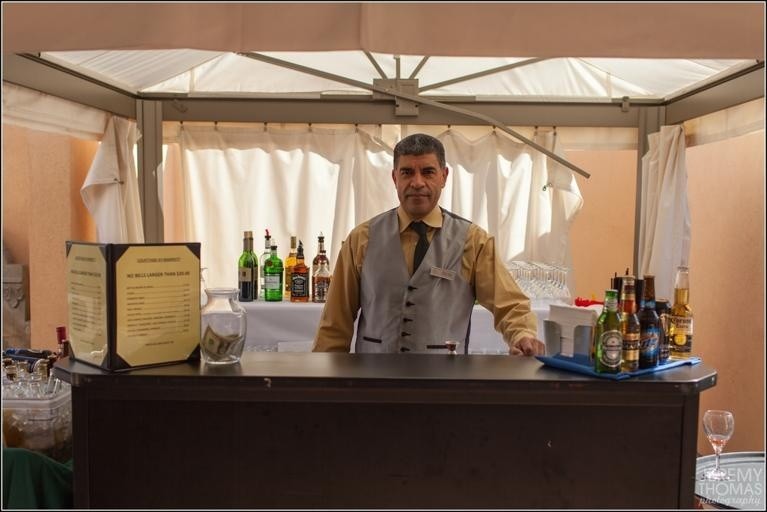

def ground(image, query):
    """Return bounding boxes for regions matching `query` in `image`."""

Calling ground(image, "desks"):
[241,300,551,354]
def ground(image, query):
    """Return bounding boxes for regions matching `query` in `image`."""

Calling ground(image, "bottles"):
[313,259,330,302]
[200,287,246,365]
[260,230,273,295]
[284,233,299,301]
[667,265,693,359]
[620,277,641,374]
[238,233,257,302]
[636,275,659,365]
[595,290,622,372]
[313,234,328,300]
[265,247,282,301]
[290,241,308,302]
[2,327,72,446]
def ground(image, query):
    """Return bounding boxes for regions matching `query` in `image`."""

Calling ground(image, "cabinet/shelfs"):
[53,355,719,509]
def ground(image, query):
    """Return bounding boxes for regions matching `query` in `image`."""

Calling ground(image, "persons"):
[310,133,546,356]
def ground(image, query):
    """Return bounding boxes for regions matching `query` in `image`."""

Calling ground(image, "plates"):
[694,451,766,510]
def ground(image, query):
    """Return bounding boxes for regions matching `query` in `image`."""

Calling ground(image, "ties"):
[409,220,430,274]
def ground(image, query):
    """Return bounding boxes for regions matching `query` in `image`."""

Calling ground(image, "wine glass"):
[510,260,571,310]
[702,410,735,480]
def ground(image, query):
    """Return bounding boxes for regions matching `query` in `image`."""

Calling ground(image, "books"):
[65,241,201,372]
